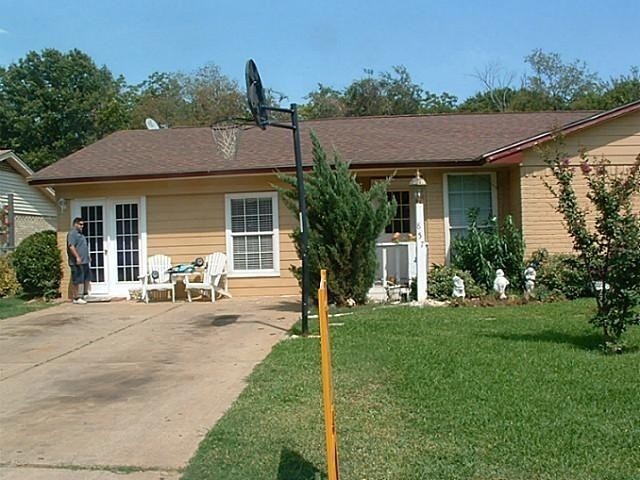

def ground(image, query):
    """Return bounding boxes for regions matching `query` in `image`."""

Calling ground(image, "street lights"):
[409,168,429,303]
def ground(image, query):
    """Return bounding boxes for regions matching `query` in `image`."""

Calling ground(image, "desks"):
[167,272,206,300]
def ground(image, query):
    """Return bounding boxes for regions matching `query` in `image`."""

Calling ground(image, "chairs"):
[137,254,176,303]
[185,252,232,302]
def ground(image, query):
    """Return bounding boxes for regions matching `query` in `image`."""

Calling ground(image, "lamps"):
[57,198,66,214]
[408,169,427,203]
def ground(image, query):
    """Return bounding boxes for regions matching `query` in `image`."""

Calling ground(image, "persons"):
[66,216,93,304]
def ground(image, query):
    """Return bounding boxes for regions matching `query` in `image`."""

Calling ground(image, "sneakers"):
[82,295,88,299]
[73,298,87,304]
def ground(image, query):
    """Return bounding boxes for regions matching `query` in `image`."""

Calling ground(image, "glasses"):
[77,222,85,226]
